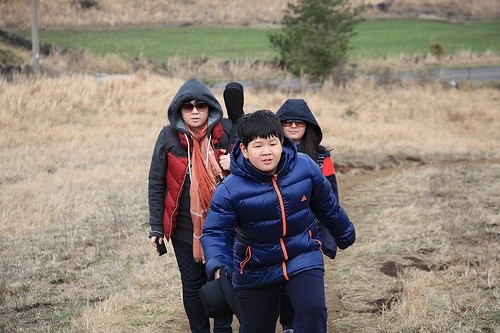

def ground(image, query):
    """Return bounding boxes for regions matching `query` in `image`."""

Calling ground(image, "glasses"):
[181,101,208,113]
[281,120,307,127]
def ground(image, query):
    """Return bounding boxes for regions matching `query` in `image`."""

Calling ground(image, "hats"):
[200,265,243,325]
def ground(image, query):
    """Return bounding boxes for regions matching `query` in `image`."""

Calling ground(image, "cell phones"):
[156,238,167,256]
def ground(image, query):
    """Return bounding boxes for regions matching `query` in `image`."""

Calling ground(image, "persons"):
[147,79,356,333]
